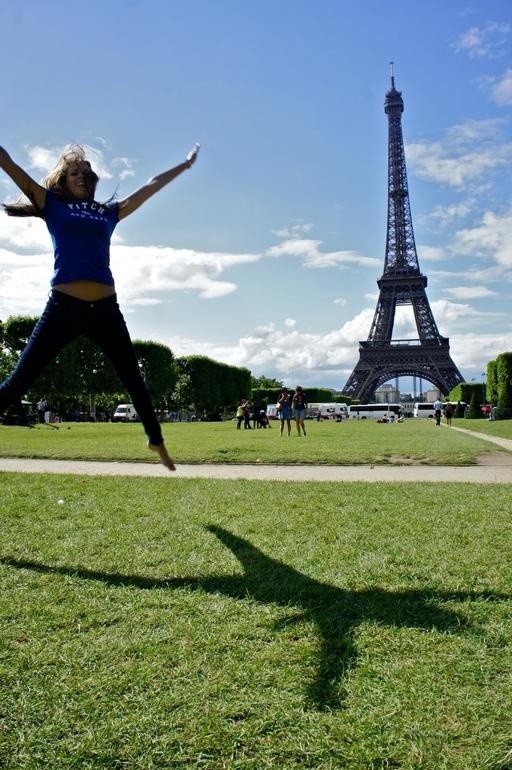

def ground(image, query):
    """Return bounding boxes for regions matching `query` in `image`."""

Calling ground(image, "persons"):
[434,398,454,428]
[382,413,404,423]
[236,399,271,429]
[37,399,46,424]
[277,385,307,437]
[0,143,200,471]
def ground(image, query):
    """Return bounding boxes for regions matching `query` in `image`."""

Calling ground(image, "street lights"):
[470,372,486,404]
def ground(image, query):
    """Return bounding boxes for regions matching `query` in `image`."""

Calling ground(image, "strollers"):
[259,414,272,429]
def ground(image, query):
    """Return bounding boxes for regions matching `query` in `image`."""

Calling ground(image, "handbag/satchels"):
[275,401,282,411]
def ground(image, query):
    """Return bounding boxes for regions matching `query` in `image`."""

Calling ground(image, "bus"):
[232,400,496,421]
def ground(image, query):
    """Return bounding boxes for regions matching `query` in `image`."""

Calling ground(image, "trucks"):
[112,402,137,422]
